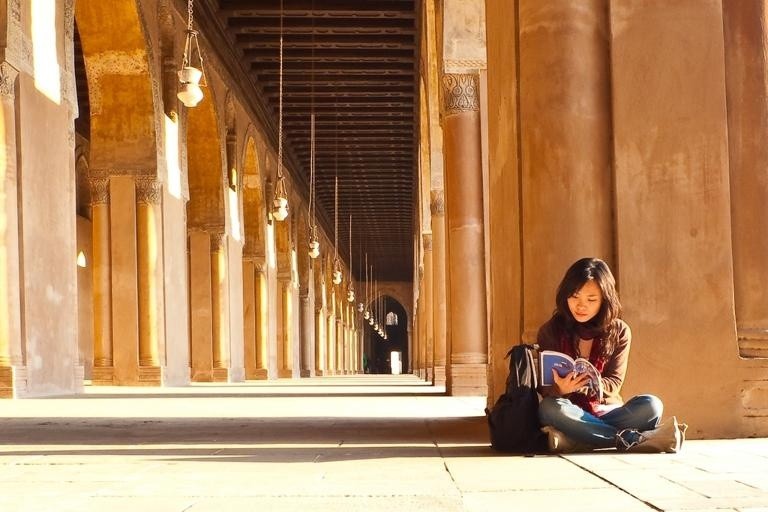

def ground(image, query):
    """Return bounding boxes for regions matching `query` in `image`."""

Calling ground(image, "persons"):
[532,256,688,453]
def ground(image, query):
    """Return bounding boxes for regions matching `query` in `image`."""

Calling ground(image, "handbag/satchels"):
[486,344,546,453]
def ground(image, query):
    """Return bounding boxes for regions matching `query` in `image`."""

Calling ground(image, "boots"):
[547,428,592,452]
[624,416,687,452]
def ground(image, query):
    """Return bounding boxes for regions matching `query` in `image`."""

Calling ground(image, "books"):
[538,348,604,403]
